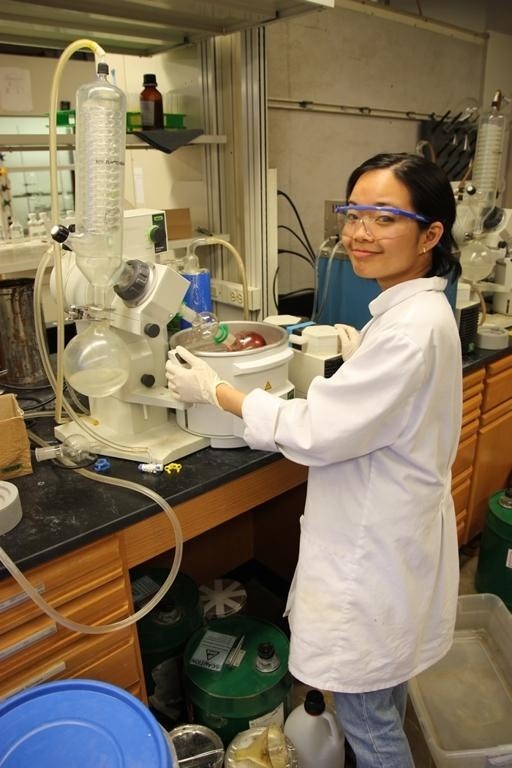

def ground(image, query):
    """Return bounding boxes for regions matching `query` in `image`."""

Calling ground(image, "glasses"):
[330,204,424,240]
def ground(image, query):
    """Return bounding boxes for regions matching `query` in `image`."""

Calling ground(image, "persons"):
[165,152,464,766]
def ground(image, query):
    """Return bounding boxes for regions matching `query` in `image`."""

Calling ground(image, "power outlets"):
[210,278,261,310]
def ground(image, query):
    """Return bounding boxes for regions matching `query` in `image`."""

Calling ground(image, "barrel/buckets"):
[181,614,295,753]
[283,689,346,768]
[0,677,174,768]
[130,565,205,695]
[474,488,512,614]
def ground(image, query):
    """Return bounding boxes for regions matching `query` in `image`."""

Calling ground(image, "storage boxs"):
[406,593,512,768]
[0,392,32,479]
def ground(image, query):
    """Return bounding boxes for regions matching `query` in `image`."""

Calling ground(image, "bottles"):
[138,72,164,129]
[10,210,74,241]
[56,100,76,133]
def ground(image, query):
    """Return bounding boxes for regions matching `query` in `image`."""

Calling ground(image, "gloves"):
[335,324,359,361]
[164,345,230,412]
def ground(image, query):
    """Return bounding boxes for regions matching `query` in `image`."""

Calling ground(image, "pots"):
[169,319,297,451]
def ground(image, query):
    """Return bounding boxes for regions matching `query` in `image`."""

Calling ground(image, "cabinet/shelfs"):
[449,370,482,547]
[3,538,144,703]
[465,356,512,539]
